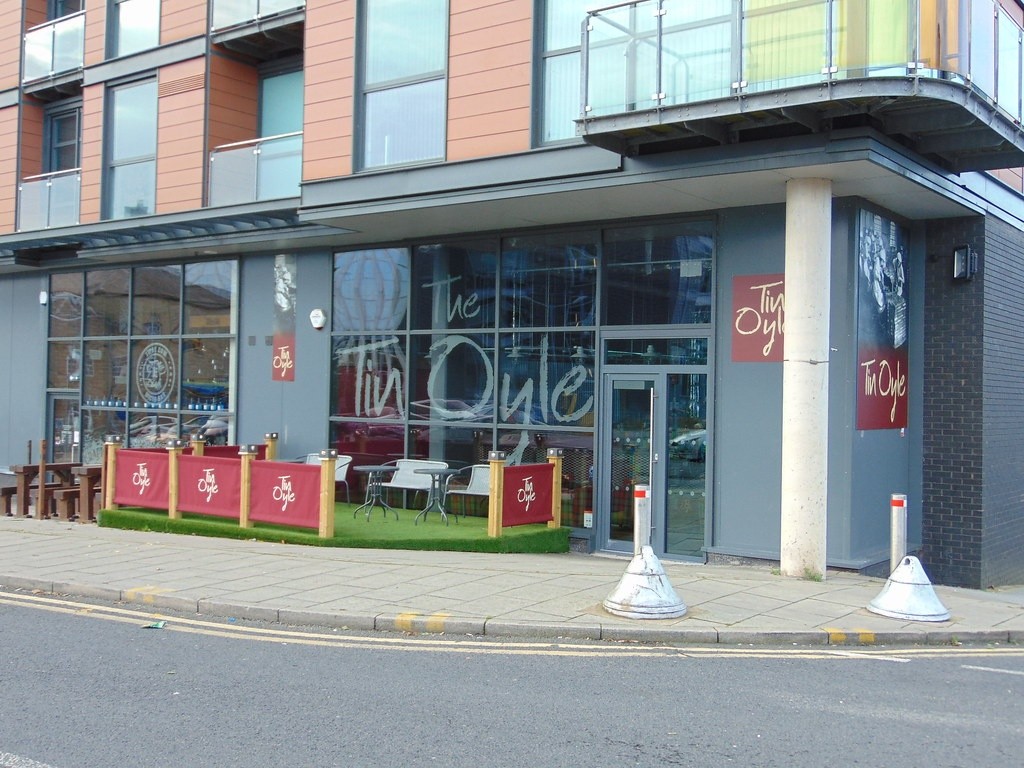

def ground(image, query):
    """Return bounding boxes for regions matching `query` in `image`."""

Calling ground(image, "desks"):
[71,467,101,523]
[351,466,399,523]
[413,469,460,526]
[9,462,83,518]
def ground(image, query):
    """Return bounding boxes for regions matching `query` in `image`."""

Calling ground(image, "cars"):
[129,415,211,438]
[673,430,706,460]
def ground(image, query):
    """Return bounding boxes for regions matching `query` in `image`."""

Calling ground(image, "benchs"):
[363,459,449,514]
[0,483,101,521]
[297,453,352,507]
[441,464,490,525]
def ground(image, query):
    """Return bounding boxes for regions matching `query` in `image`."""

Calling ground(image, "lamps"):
[953,242,977,281]
